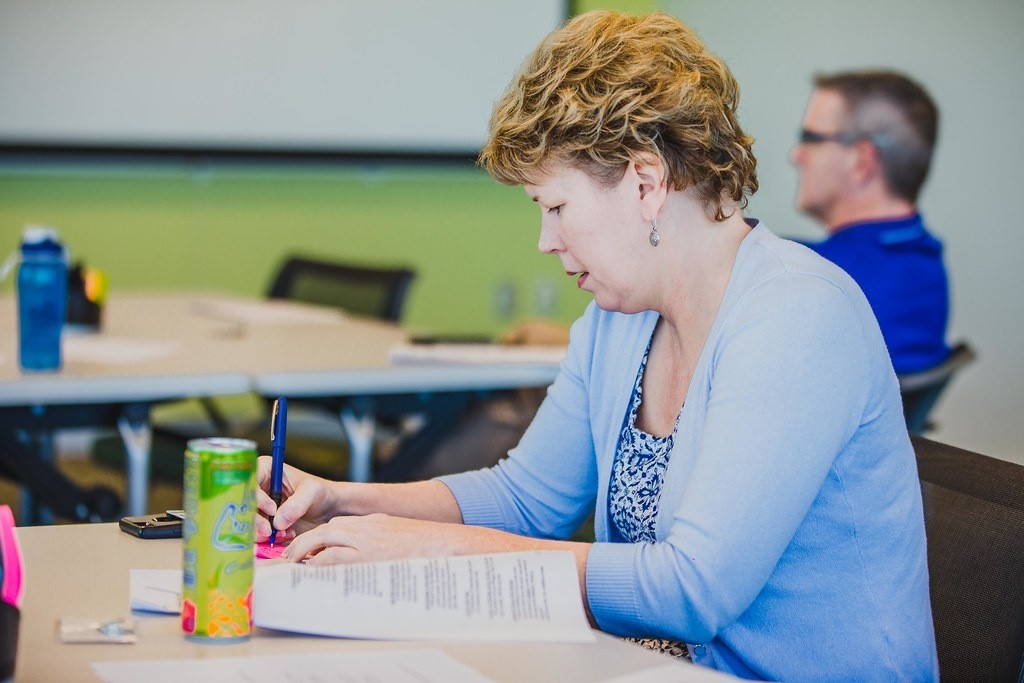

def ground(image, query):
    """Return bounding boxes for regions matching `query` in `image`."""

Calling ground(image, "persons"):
[252,9,943,683]
[377,71,948,484]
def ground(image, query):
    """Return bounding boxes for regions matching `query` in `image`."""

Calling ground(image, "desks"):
[16,522,754,683]
[0,294,568,525]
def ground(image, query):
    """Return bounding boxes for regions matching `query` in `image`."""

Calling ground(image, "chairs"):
[268,258,417,325]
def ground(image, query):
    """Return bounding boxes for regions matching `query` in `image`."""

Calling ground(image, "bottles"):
[16,230,67,369]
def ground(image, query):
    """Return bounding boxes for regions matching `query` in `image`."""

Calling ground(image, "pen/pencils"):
[269,396,288,547]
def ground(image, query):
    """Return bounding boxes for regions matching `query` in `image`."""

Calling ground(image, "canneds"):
[184,438,258,640]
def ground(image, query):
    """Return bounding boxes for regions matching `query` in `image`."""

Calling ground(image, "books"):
[134,552,592,638]
